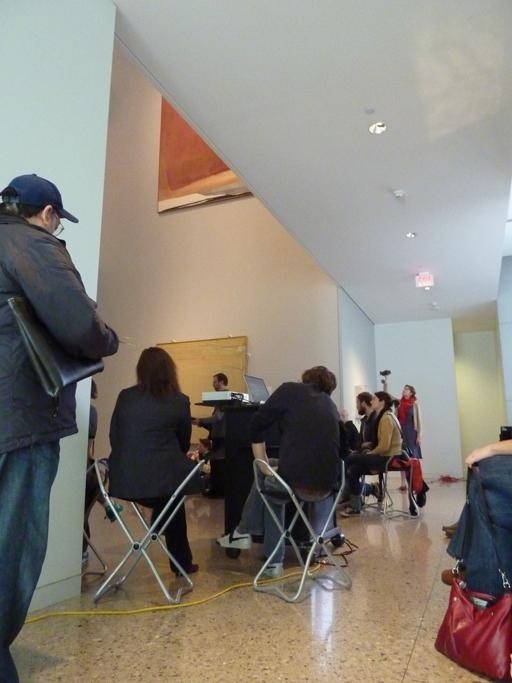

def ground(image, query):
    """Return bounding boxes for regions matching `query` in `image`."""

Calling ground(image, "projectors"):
[202,390,244,401]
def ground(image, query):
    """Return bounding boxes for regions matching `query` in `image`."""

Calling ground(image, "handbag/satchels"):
[5,282,108,401]
[400,435,414,458]
[433,570,512,683]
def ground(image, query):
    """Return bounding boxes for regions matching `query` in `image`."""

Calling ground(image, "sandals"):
[398,483,407,492]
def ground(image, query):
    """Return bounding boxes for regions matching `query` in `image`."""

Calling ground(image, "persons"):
[185,437,213,477]
[395,383,423,463]
[211,360,340,579]
[189,371,231,500]
[339,390,405,517]
[79,377,124,570]
[106,345,201,575]
[0,170,131,683]
[441,425,510,605]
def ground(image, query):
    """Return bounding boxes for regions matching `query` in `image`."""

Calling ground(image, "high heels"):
[174,562,200,578]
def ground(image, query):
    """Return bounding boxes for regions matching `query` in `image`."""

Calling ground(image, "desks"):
[218,401,342,548]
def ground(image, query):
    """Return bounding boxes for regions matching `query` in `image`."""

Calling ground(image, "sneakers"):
[339,507,362,518]
[261,560,284,580]
[215,525,253,550]
[441,520,461,540]
[369,481,383,503]
[440,567,467,586]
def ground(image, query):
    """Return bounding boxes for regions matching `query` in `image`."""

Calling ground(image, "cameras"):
[380,369,391,376]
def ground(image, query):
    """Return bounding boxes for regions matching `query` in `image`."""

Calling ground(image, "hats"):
[2,173,80,224]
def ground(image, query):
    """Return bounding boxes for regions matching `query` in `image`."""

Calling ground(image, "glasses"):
[51,208,66,238]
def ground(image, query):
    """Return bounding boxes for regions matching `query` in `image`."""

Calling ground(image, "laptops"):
[242,374,270,404]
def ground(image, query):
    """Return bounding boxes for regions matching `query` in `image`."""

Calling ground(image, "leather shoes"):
[104,500,125,523]
[82,551,89,564]
